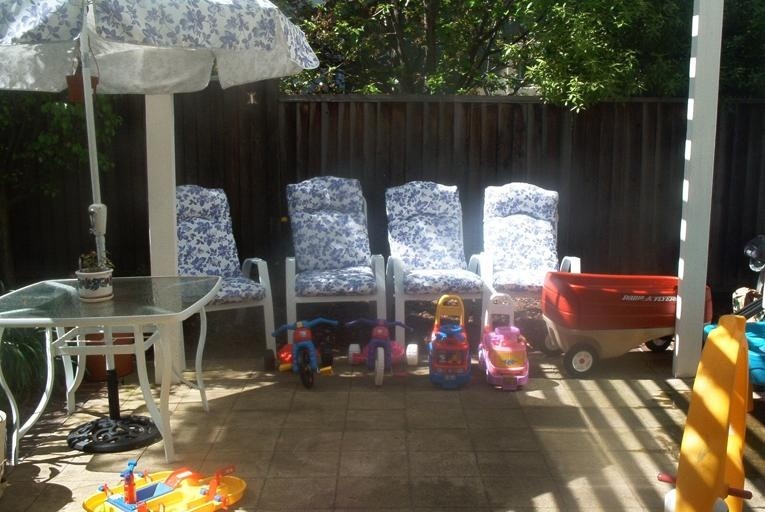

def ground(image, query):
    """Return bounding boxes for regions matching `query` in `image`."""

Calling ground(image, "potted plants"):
[75,246,115,302]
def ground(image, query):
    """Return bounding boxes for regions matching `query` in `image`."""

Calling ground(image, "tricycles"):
[343,315,420,387]
[262,317,340,390]
[537,254,714,379]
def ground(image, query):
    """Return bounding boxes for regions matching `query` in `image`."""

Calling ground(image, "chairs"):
[174,183,279,373]
[469,181,583,352]
[284,176,387,386]
[384,179,482,368]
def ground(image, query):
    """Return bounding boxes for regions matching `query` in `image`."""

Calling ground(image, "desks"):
[0,273,223,464]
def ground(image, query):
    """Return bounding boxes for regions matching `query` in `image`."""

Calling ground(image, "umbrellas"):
[0,0,318,417]
